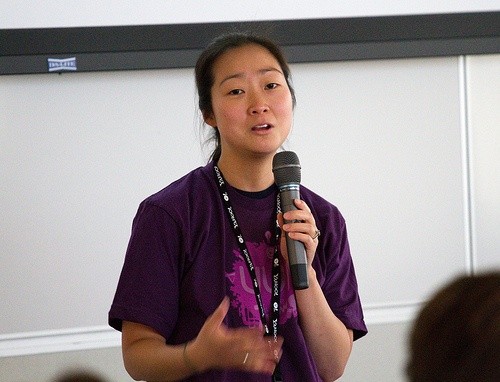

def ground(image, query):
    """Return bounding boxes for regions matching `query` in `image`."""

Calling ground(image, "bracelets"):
[182,342,200,375]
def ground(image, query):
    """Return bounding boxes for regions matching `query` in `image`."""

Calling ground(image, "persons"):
[107,35,368,382]
[404,268,500,382]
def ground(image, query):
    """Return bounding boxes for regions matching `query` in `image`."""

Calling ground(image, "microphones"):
[272,150,309,290]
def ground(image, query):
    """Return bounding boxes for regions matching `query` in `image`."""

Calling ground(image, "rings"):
[273,350,279,360]
[311,229,321,239]
